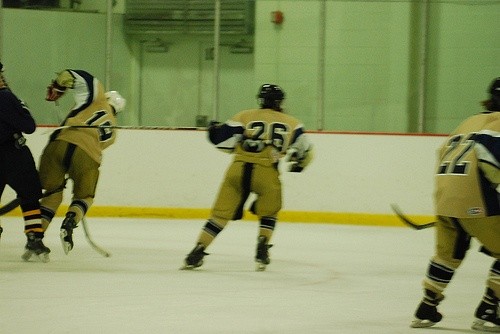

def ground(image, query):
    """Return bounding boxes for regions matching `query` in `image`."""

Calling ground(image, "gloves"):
[289,164,302,172]
[46,85,65,102]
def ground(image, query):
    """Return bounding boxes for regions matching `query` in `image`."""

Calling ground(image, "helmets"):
[104,90,126,116]
[257,83,284,109]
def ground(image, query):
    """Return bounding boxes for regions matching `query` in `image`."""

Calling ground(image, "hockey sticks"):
[390,203,435,231]
[50,126,209,133]
[56,102,110,258]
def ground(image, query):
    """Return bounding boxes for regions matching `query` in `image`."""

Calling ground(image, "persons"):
[415,76,500,326]
[24,68,126,253]
[185,83,313,267]
[0,62,51,255]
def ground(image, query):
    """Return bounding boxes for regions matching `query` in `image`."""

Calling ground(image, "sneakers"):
[26,234,51,261]
[181,243,209,270]
[410,290,445,327]
[60,211,77,255]
[255,237,273,270]
[474,287,500,331]
[22,250,32,262]
